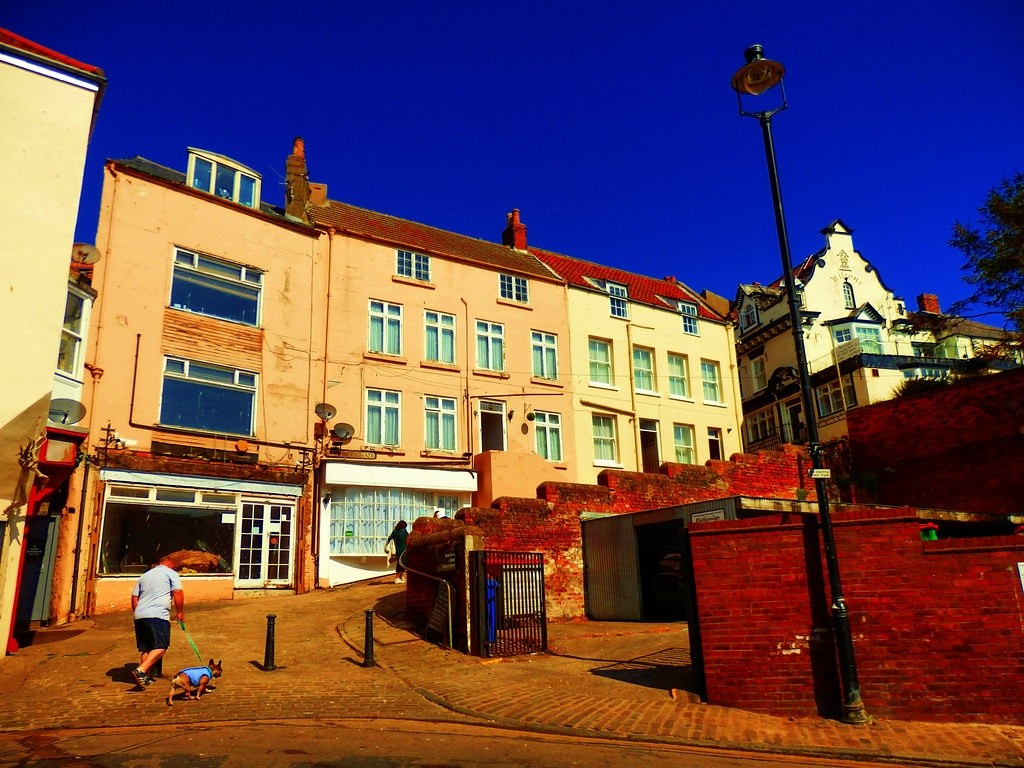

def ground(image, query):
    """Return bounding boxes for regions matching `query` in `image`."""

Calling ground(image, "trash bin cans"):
[487,578,500,643]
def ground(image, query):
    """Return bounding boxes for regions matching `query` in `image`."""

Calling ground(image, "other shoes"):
[395,576,406,584]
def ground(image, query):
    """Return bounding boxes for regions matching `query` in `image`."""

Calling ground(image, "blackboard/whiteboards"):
[427,582,459,632]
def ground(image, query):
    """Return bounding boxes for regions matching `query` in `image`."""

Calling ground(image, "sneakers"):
[132,667,156,691]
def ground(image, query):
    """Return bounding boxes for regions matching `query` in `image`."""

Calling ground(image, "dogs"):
[169,658,222,706]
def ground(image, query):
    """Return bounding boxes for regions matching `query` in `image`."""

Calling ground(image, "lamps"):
[324,488,332,505]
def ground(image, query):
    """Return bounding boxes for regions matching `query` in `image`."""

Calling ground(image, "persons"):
[384,520,409,584]
[132,556,185,691]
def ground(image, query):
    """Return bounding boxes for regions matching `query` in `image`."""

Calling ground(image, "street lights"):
[729,42,870,725]
